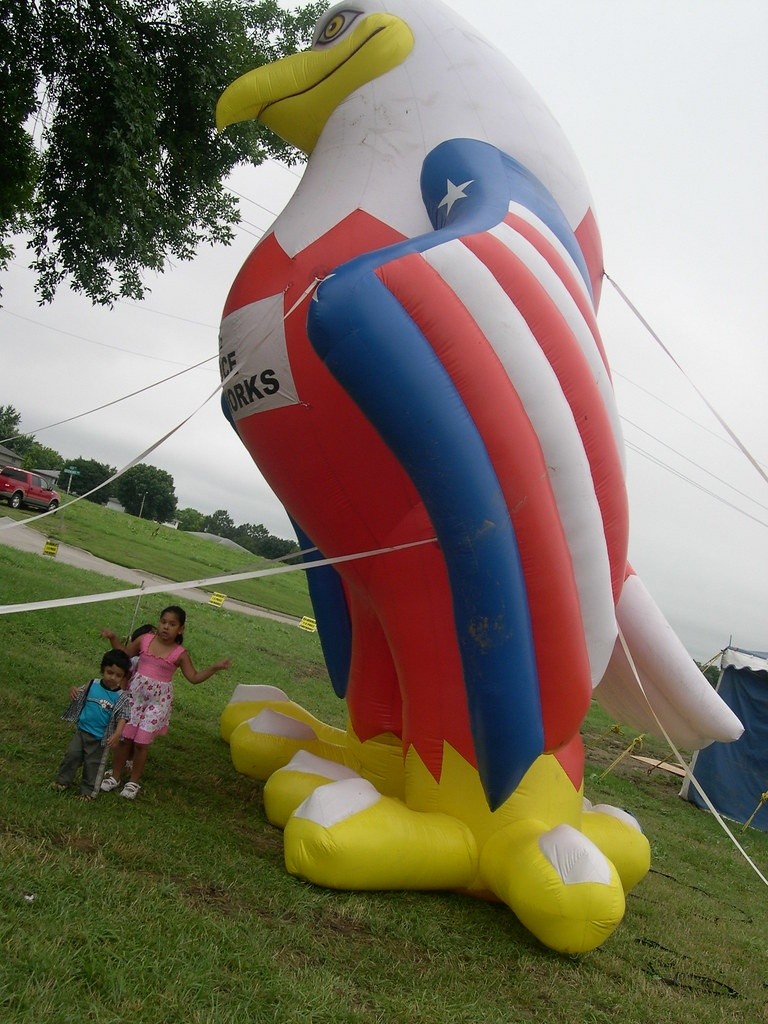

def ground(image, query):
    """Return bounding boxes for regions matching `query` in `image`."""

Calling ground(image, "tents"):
[679,645,768,834]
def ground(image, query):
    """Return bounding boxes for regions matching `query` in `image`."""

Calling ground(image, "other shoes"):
[80,792,94,803]
[120,782,141,799]
[53,782,73,791]
[100,776,121,792]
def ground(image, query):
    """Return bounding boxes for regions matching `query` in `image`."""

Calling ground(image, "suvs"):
[0,467,61,515]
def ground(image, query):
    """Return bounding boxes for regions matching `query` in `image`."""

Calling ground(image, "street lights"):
[139,492,148,518]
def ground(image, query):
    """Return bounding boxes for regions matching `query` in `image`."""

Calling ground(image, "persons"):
[101,606,229,800]
[52,648,130,799]
